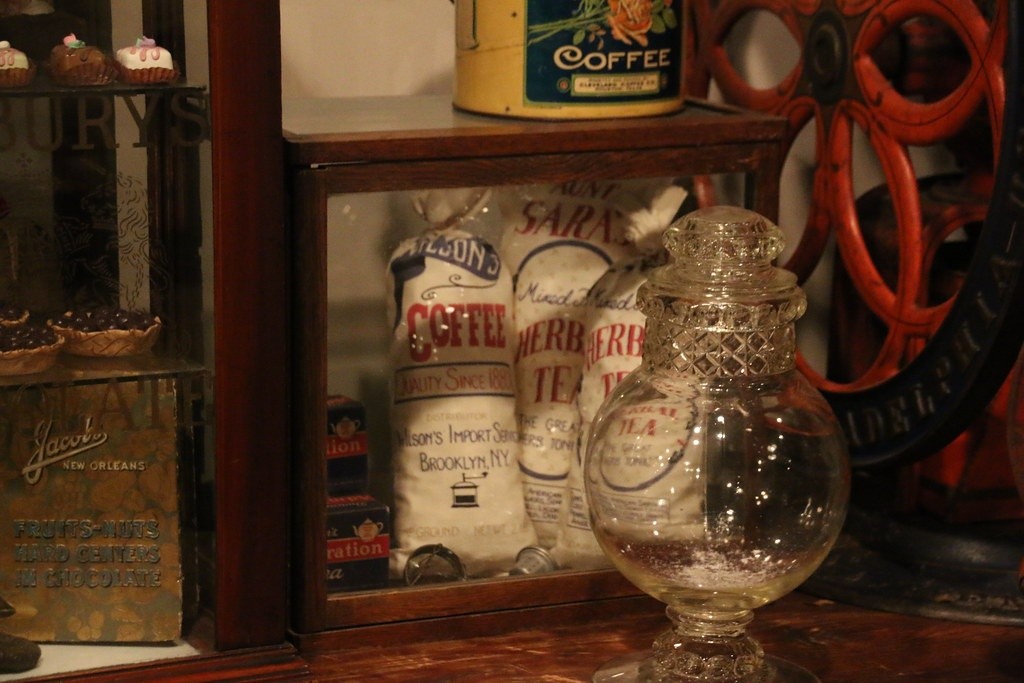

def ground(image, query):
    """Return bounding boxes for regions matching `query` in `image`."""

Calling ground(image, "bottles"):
[577,206,850,683]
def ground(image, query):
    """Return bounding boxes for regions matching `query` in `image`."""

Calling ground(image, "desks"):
[306,592,1024,683]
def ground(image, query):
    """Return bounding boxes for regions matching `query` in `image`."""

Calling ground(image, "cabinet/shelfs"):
[0,0,799,683]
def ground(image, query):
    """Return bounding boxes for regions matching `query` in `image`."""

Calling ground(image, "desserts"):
[0,33,181,87]
[0,301,163,375]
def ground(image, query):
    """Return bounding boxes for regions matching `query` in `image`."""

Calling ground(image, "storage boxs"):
[327,394,390,595]
